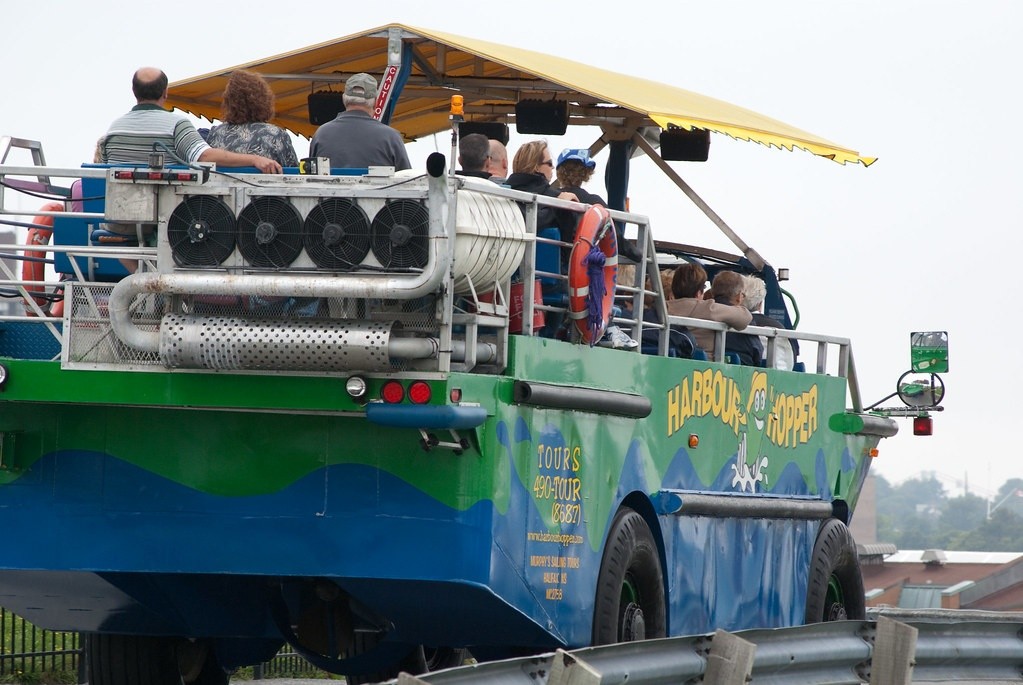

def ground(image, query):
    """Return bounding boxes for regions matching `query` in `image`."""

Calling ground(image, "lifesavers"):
[21,201,73,317]
[568,203,619,345]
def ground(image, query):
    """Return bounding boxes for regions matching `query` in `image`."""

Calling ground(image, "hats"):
[557,148,596,171]
[344,73,378,100]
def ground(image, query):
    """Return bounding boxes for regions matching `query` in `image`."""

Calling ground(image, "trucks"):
[0,21,950,685]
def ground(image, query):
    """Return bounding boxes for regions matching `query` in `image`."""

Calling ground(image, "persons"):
[99,67,284,174]
[614,264,696,358]
[456,133,580,233]
[711,272,786,367]
[70,137,104,212]
[506,140,581,296]
[487,139,511,188]
[206,70,300,167]
[309,74,412,171]
[747,276,799,362]
[667,264,753,364]
[555,149,643,263]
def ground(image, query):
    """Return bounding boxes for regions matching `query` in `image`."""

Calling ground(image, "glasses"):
[537,159,552,167]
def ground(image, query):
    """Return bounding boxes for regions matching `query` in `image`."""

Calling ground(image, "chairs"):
[52,162,806,372]
